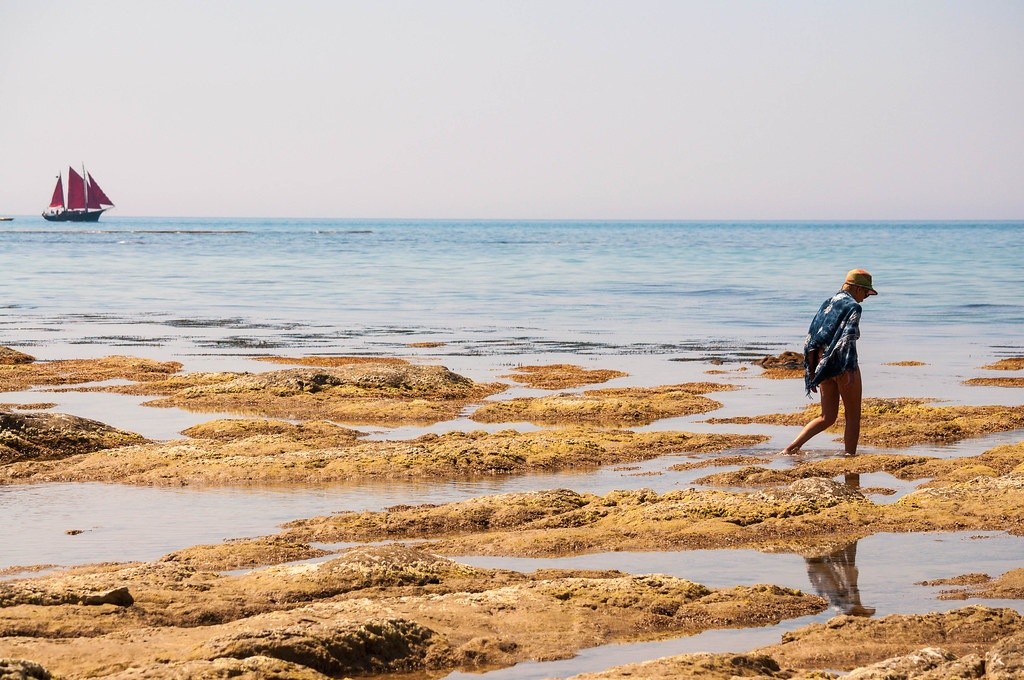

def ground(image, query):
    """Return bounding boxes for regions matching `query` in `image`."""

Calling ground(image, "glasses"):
[863,288,871,299]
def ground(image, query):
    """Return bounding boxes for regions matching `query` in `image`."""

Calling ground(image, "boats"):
[0,216,14,221]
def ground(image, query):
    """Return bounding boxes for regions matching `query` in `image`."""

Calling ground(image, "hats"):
[845,269,878,295]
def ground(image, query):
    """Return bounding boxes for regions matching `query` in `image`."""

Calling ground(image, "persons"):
[780,269,879,455]
[806,474,876,617]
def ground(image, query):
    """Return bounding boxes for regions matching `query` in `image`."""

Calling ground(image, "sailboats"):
[43,160,116,223]
